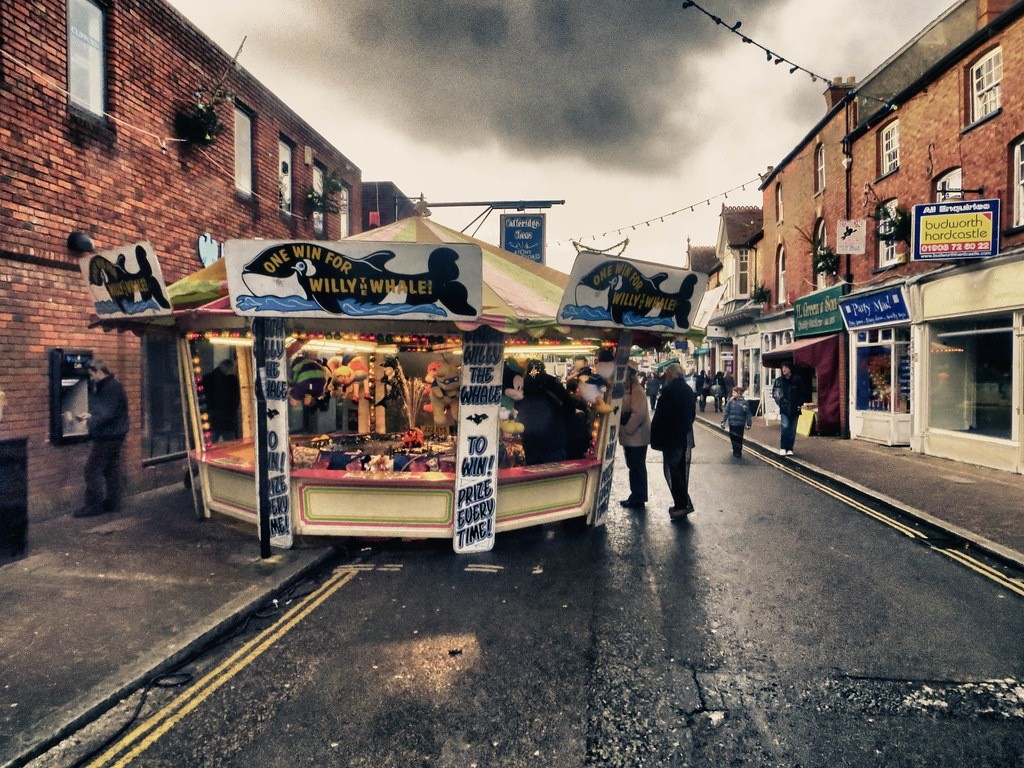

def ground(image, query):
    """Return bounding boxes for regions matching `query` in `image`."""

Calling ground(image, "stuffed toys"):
[287,347,615,464]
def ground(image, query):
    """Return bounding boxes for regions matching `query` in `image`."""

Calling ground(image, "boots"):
[719,397,722,412]
[700,400,706,411]
[714,398,718,412]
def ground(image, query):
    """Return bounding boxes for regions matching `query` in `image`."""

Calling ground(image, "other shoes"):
[102,499,121,512]
[75,503,103,516]
[620,500,644,508]
[787,450,793,455]
[669,506,695,519]
[733,452,741,457]
[780,449,786,456]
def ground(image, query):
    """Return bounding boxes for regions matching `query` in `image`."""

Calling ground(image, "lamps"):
[67,232,96,252]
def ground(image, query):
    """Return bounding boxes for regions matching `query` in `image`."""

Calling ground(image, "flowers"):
[871,206,911,247]
[304,169,349,219]
[175,99,227,149]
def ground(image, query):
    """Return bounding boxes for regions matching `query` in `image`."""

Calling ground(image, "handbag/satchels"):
[620,412,641,428]
[702,381,710,388]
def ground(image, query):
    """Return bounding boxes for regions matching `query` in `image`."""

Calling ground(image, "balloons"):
[867,354,891,401]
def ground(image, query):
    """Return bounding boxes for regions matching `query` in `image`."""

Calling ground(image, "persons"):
[651,363,696,519]
[638,372,662,409]
[721,387,752,456]
[617,360,651,507]
[696,370,734,413]
[773,361,805,455]
[73,359,129,518]
[685,367,698,401]
[184,360,240,488]
